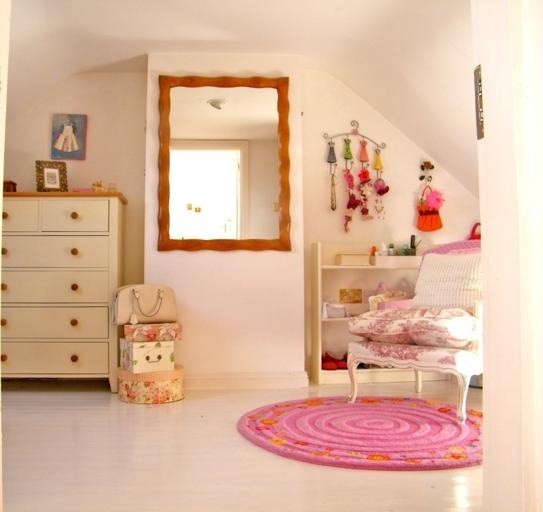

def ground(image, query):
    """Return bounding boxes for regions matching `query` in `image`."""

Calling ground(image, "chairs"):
[346,239,483,421]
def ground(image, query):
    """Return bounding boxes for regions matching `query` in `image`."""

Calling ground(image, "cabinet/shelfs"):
[311,242,451,385]
[0,192,127,395]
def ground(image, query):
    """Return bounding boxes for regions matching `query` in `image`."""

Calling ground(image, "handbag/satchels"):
[416,204,443,232]
[112,284,178,327]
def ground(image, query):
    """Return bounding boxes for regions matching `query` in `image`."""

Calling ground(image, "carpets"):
[237,395,483,470]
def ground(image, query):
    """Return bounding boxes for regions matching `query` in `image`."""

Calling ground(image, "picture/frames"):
[34,161,66,191]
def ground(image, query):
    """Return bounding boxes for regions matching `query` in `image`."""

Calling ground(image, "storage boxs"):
[116,323,184,404]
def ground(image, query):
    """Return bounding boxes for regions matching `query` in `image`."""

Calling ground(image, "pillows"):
[348,252,479,349]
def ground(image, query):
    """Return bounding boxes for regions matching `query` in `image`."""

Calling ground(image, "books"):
[333,252,371,266]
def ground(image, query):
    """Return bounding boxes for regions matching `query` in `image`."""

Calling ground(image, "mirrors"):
[156,75,290,252]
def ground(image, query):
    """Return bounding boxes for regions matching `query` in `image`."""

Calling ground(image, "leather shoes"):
[322,351,347,369]
